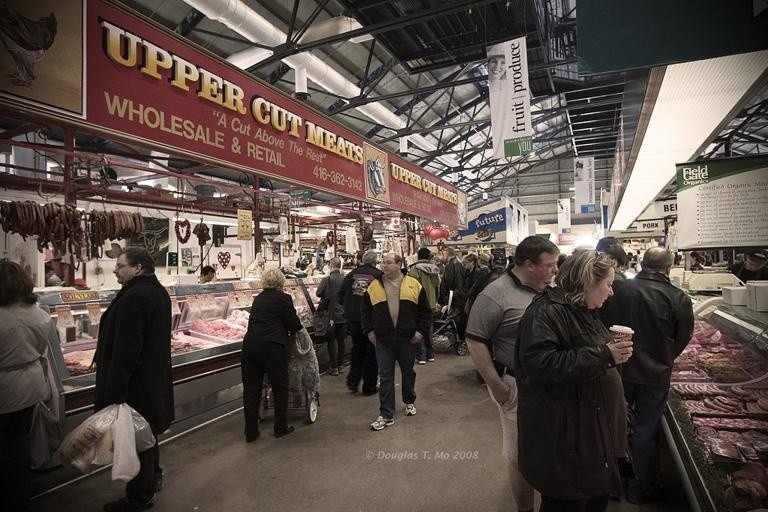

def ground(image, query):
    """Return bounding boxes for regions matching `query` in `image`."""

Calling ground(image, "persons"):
[95,246,178,510]
[1,258,51,511]
[196,265,216,283]
[45,266,66,286]
[240,236,768,511]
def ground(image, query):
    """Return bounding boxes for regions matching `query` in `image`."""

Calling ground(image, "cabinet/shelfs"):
[593,259,766,512]
[1,254,449,510]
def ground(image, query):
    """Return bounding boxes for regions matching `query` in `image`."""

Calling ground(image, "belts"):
[498,365,513,376]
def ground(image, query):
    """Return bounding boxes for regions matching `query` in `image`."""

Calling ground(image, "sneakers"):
[326,366,339,376]
[405,403,417,415]
[275,426,295,438]
[370,416,394,430]
[246,430,261,442]
[426,357,434,362]
[414,357,426,364]
[338,365,344,372]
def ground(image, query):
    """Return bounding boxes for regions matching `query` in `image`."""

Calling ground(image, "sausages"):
[672,384,768,415]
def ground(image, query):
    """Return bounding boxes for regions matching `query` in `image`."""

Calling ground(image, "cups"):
[608,325,635,350]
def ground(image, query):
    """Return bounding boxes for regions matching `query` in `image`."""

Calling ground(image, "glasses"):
[595,250,605,263]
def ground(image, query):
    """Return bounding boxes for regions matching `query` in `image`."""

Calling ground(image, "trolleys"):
[259,310,325,424]
[432,305,467,356]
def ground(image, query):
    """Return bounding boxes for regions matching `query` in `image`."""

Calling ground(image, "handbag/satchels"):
[312,312,335,338]
